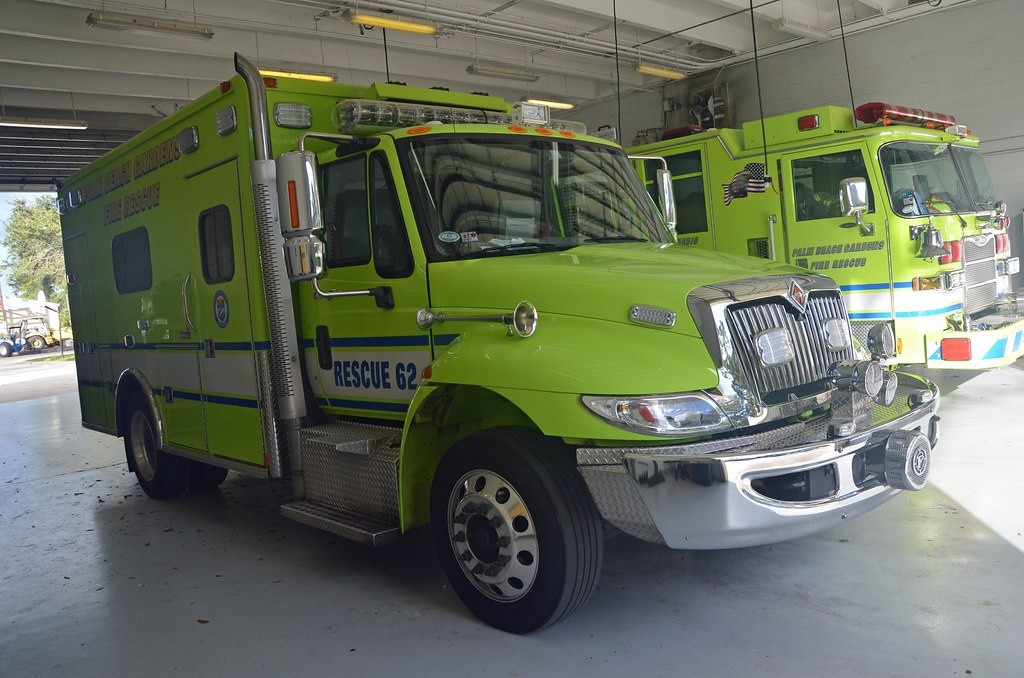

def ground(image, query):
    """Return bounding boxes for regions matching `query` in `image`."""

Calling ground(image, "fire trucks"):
[53,52,942,636]
[625,102,1023,370]
